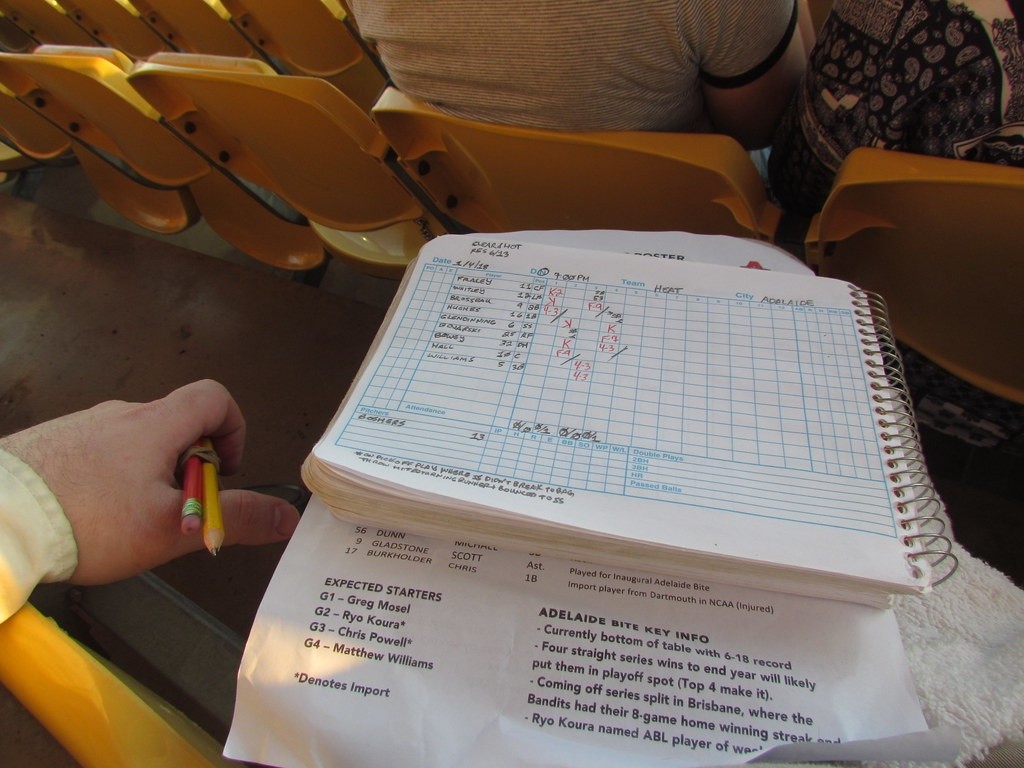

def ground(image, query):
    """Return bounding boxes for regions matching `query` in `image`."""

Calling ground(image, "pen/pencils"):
[203,440,225,557]
[180,440,203,535]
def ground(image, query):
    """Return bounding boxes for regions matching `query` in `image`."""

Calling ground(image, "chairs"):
[0,0,780,326]
[800,148,1024,448]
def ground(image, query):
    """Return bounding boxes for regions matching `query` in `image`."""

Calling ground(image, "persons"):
[346,0,817,205]
[0,378,300,627]
[767,0,1024,212]
